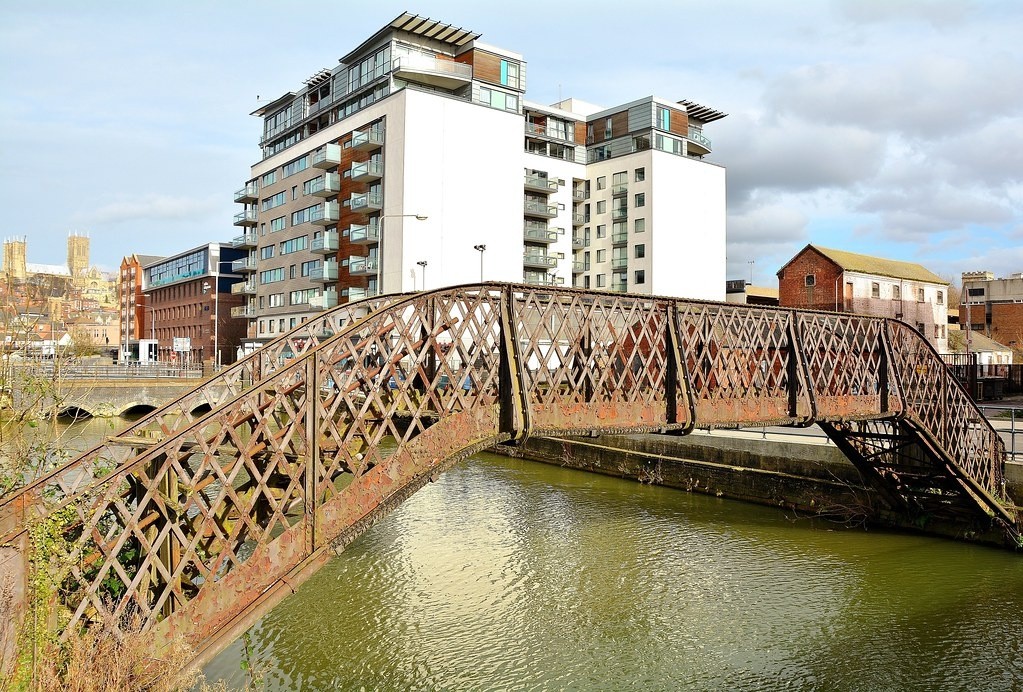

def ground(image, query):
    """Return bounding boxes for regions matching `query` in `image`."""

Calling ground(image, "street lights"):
[417,261,428,291]
[376,214,428,394]
[474,244,486,327]
[136,304,155,367]
[214,261,244,372]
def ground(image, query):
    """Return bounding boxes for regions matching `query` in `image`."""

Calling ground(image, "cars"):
[371,368,406,390]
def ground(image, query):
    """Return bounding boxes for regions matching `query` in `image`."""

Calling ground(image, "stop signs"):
[171,352,176,359]
